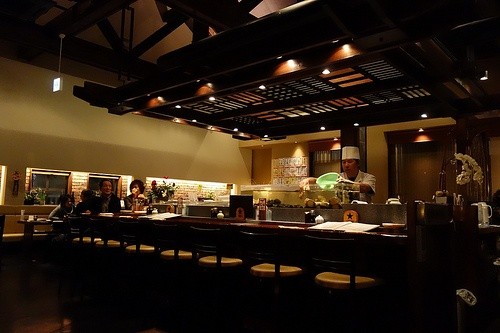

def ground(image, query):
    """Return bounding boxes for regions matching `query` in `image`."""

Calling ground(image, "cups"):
[21,210,25,215]
[34,215,38,221]
[29,215,34,220]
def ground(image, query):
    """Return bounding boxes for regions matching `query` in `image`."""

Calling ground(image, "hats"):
[342,147,360,160]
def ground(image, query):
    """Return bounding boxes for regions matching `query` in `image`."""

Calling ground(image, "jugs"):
[472,201,492,225]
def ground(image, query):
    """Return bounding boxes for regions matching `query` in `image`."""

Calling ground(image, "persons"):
[299,146,376,203]
[49,180,149,234]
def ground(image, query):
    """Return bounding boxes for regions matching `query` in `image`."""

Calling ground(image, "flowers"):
[439,153,483,189]
[25,187,47,204]
[151,175,178,201]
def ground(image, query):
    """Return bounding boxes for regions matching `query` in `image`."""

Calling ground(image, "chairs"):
[59,217,375,333]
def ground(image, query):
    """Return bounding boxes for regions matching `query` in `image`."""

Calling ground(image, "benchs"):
[0,204,58,242]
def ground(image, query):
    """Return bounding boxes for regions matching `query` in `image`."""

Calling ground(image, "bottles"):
[211,207,224,218]
[304,210,325,223]
[146,205,159,214]
[176,204,186,216]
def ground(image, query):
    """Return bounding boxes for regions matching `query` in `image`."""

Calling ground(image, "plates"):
[120,209,146,213]
[382,222,406,229]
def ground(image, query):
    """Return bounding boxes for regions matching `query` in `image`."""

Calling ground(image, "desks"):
[17,220,53,266]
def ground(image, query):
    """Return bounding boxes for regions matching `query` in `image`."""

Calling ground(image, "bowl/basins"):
[316,172,341,191]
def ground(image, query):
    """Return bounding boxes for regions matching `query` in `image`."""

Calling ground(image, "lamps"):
[53,34,65,93]
